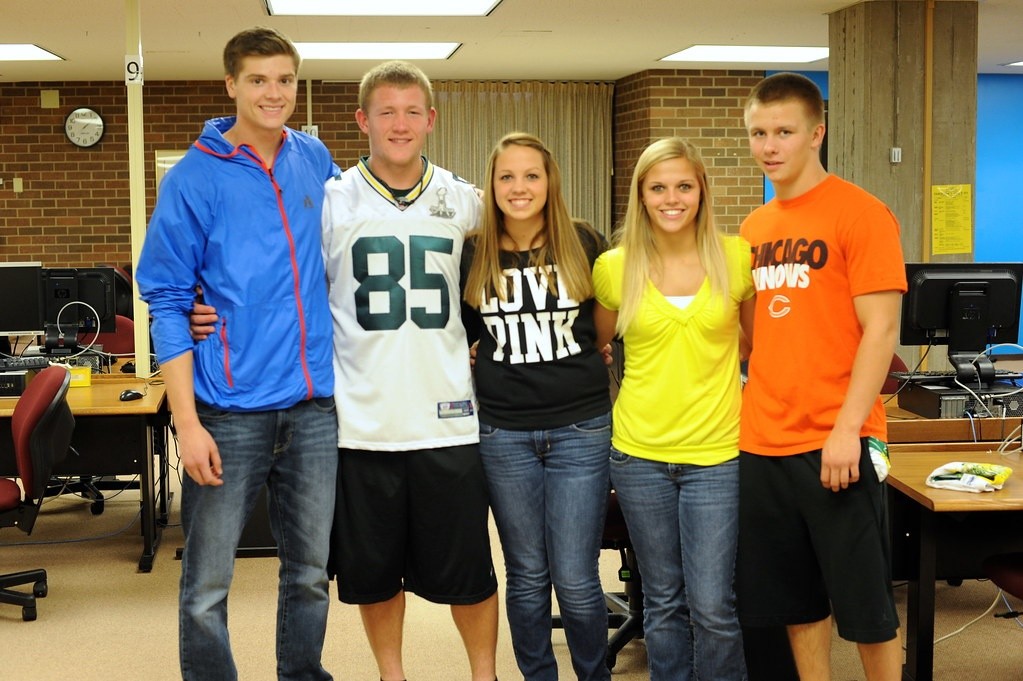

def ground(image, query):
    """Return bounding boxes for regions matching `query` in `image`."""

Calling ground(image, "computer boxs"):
[22,344,104,374]
[897,380,1023,420]
[0,370,30,399]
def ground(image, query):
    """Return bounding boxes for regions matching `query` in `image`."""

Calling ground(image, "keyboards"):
[889,368,1023,383]
[0,358,48,371]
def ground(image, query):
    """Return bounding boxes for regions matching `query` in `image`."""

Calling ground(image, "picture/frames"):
[155,149,188,197]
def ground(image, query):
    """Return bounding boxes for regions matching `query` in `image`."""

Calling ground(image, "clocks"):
[64,106,106,149]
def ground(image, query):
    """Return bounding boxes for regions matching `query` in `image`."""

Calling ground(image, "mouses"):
[120,389,143,401]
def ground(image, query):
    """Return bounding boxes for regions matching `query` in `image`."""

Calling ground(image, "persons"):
[188,61,616,681]
[459,132,613,681]
[734,72,907,681]
[136,27,343,681]
[469,137,757,681]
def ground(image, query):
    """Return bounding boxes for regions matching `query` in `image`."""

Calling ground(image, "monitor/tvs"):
[0,262,116,354]
[899,262,1023,388]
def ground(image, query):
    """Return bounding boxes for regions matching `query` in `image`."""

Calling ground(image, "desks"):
[879,394,1023,443]
[91,357,174,530]
[886,443,1023,681]
[0,377,167,573]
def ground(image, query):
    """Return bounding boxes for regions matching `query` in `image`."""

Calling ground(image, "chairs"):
[551,484,644,672]
[44,315,140,518]
[0,366,76,621]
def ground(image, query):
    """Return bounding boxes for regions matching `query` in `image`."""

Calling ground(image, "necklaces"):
[366,157,426,207]
